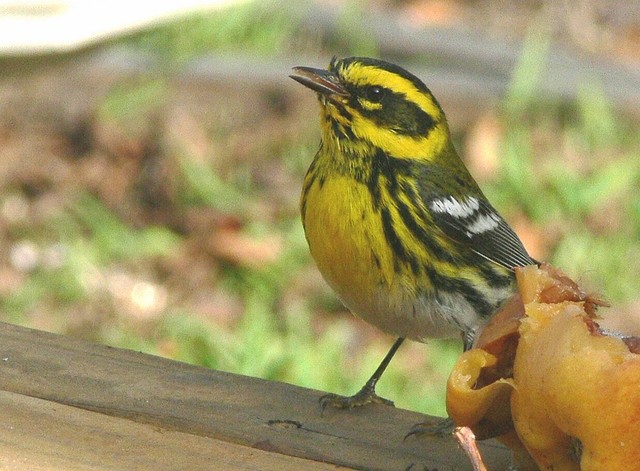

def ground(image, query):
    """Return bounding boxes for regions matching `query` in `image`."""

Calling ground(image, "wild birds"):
[287,58,541,442]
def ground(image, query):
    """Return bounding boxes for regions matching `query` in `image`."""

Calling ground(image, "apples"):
[445,263,640,470]
